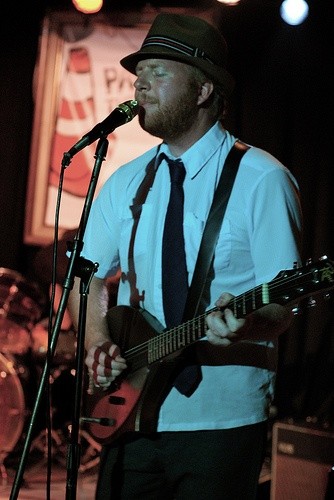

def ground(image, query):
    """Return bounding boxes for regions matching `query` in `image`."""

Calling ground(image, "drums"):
[1,354,43,471]
[0,268,44,354]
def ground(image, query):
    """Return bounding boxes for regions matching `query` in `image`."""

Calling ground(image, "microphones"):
[63,100,140,165]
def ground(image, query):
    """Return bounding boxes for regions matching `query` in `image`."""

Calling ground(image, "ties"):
[159,157,203,398]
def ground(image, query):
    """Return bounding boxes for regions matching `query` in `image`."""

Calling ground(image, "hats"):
[120,13,236,92]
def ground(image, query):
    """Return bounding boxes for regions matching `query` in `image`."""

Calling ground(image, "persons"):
[66,14,306,500]
[3,278,89,472]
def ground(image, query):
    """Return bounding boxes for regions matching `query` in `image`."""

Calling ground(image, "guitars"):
[80,253,334,439]
